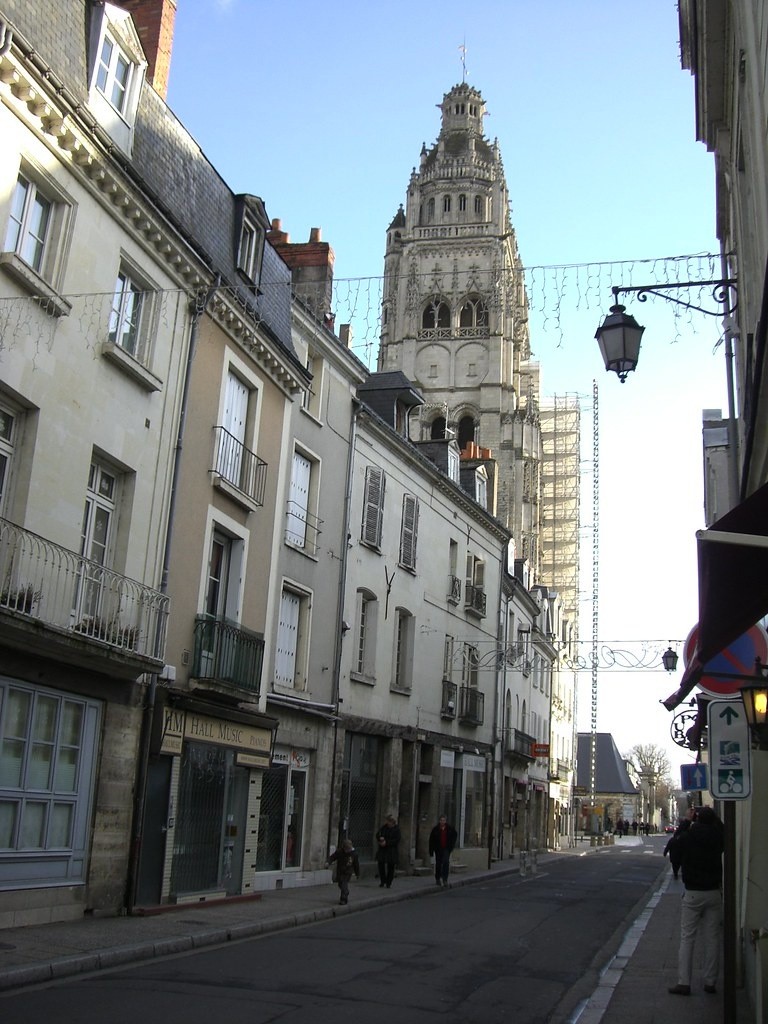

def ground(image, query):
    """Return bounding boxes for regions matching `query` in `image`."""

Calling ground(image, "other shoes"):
[386,883,390,888]
[443,880,448,886]
[436,879,442,885]
[669,984,691,996]
[340,900,347,905]
[703,985,715,992]
[380,882,386,886]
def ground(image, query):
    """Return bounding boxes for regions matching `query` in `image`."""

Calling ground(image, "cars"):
[665,822,676,832]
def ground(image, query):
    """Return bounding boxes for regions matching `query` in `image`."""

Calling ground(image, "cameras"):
[694,806,705,812]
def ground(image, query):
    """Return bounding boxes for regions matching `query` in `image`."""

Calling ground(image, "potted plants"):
[75,609,147,651]
[0,582,43,614]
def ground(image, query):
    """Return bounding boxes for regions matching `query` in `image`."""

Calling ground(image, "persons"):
[624,819,629,835]
[645,823,650,835]
[639,822,644,835]
[631,820,638,835]
[324,837,360,905]
[428,814,458,887]
[667,806,727,997]
[663,808,702,880]
[376,814,401,888]
[616,818,624,838]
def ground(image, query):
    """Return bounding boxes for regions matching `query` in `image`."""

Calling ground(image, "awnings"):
[660,481,768,711]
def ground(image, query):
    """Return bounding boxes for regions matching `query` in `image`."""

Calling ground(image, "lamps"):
[736,656,768,751]
[593,279,739,384]
[662,646,679,672]
